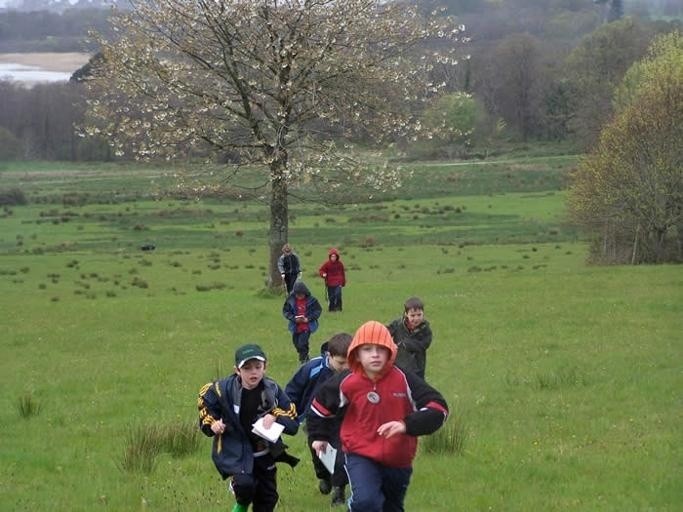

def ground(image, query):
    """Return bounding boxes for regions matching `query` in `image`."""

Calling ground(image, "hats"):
[235,344,266,369]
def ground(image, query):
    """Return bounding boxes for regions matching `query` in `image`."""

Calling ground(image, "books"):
[318,442,337,475]
[294,315,306,320]
[250,417,285,444]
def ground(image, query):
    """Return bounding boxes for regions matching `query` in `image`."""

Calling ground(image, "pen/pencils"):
[220,417,223,434]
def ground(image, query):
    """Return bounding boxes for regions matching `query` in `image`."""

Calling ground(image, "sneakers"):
[232,502,248,512]
[320,479,332,495]
[333,487,345,508]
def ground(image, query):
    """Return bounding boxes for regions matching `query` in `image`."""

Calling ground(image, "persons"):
[276,243,302,294]
[195,344,300,512]
[282,282,323,365]
[317,249,347,311]
[306,318,450,512]
[380,296,433,382]
[284,329,361,509]
